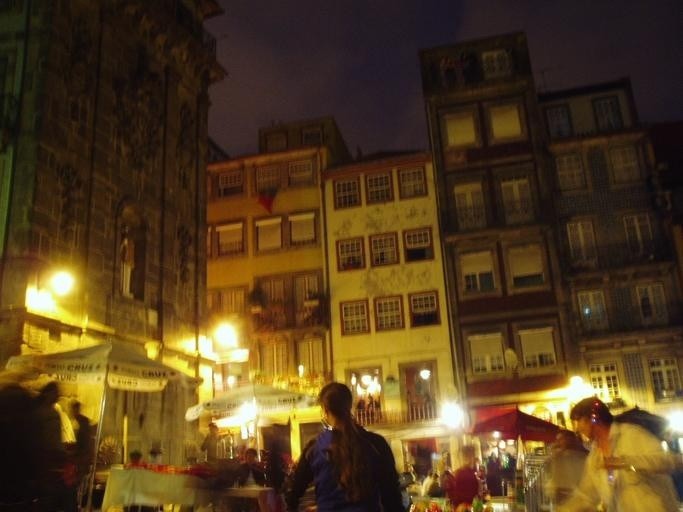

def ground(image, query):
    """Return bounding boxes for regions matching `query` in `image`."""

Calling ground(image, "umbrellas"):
[5,336,204,512]
[184,373,322,461]
[471,407,560,442]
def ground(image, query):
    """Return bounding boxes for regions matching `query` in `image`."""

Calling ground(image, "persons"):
[282,381,406,510]
[119,223,137,298]
[0,379,96,512]
[202,421,318,512]
[541,394,682,512]
[398,452,517,512]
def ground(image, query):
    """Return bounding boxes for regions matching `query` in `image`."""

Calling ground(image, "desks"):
[99,464,214,512]
[213,485,278,511]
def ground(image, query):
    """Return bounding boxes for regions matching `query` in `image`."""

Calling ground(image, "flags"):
[254,187,278,215]
